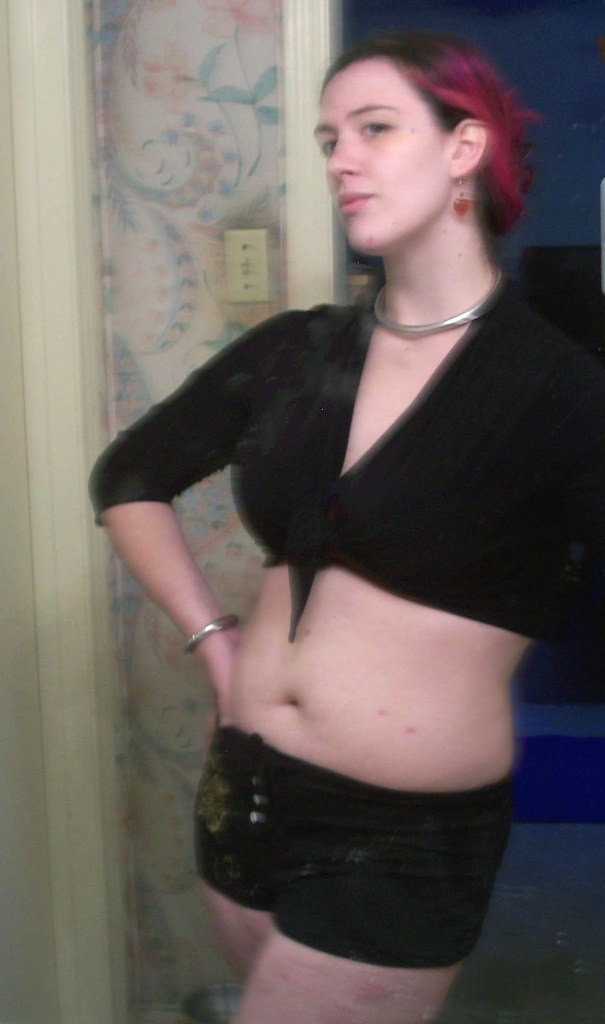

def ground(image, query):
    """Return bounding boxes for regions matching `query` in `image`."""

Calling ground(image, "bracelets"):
[186,614,239,652]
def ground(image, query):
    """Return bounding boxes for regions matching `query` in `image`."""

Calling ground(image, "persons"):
[89,34,605,1024]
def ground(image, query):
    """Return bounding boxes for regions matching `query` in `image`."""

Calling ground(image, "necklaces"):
[373,266,506,334]
